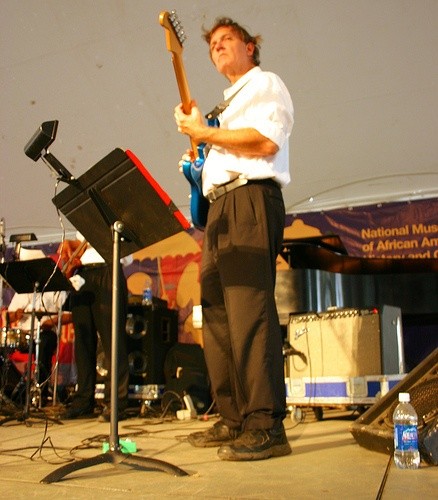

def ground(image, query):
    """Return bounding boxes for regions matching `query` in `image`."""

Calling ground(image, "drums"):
[0,355,11,393]
[1,326,31,348]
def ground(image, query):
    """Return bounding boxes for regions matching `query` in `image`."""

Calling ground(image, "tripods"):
[0,232,77,426]
[25,121,190,483]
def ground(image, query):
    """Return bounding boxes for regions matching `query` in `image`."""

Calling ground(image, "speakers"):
[349,347,438,465]
[125,304,178,417]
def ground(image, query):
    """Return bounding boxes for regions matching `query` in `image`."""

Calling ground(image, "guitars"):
[159,10,221,232]
[47,238,88,279]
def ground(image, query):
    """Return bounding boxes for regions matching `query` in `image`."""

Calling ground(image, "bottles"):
[142,280,152,306]
[393,392,420,470]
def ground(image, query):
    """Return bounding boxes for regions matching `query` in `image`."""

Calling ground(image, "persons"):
[174,18,292,461]
[0,289,74,407]
[58,228,130,421]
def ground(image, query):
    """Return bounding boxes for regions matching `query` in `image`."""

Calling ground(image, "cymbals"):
[41,313,72,327]
